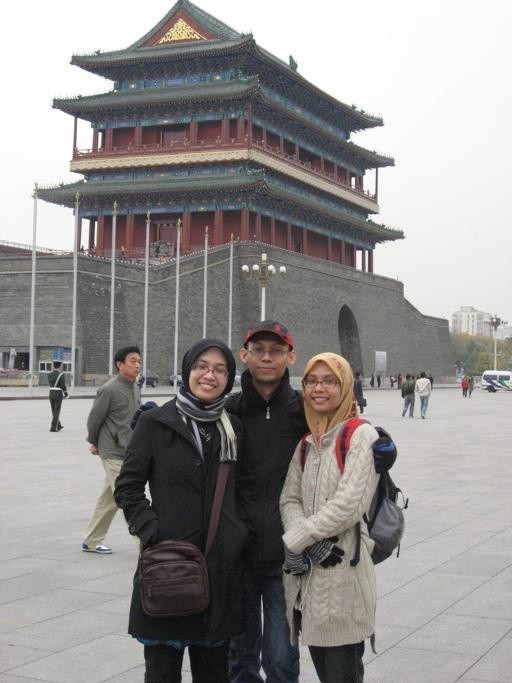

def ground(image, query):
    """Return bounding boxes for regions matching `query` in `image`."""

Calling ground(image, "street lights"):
[242,253,287,322]
[483,311,508,370]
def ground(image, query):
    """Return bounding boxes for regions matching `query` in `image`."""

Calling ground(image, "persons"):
[80,346,142,554]
[130,318,393,683]
[80,245,84,254]
[280,352,381,683]
[113,337,240,683]
[47,361,68,432]
[468,376,474,398]
[461,375,468,398]
[353,372,433,419]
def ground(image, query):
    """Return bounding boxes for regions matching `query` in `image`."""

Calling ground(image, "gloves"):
[305,534,345,568]
[373,426,399,474]
[282,543,310,577]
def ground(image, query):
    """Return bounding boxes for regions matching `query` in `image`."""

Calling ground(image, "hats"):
[53,360,63,364]
[240,319,294,349]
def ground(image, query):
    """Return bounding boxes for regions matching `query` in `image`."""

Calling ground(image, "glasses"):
[303,377,341,387]
[248,346,290,356]
[189,361,228,377]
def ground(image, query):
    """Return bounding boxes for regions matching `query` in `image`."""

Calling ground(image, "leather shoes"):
[49,427,58,432]
[58,425,64,431]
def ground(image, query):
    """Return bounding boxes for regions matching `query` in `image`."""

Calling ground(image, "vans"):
[481,370,512,392]
[232,370,241,386]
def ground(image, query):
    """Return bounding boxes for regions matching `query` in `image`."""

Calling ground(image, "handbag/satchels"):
[136,538,211,618]
[356,397,367,406]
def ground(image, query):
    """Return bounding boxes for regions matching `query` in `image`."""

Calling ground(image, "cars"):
[138,368,159,388]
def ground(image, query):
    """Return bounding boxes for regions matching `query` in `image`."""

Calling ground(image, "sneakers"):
[81,541,114,554]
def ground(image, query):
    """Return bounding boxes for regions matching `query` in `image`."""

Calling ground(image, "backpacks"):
[364,470,406,567]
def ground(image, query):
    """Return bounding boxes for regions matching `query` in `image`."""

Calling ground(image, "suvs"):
[170,372,182,385]
